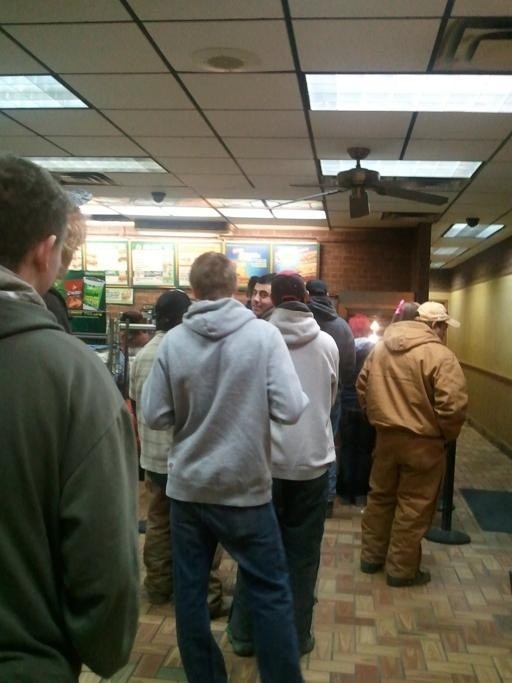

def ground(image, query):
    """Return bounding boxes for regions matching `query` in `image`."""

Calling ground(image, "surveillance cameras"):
[466,217,480,227]
[152,193,166,203]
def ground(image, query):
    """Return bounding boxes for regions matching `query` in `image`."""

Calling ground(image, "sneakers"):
[299,629,315,656]
[226,623,254,657]
[360,558,430,586]
[216,595,234,616]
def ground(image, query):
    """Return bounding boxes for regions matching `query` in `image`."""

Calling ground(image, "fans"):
[269,146,449,219]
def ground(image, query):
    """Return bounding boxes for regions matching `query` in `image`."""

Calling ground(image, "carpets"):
[459,485,511,534]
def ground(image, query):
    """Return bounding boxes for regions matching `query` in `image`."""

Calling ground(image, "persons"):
[43,204,89,332]
[356,302,469,586]
[0,150,140,683]
[128,252,356,683]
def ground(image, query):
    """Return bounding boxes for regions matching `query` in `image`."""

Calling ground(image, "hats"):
[153,290,191,330]
[306,280,327,296]
[414,301,461,328]
[272,271,305,306]
